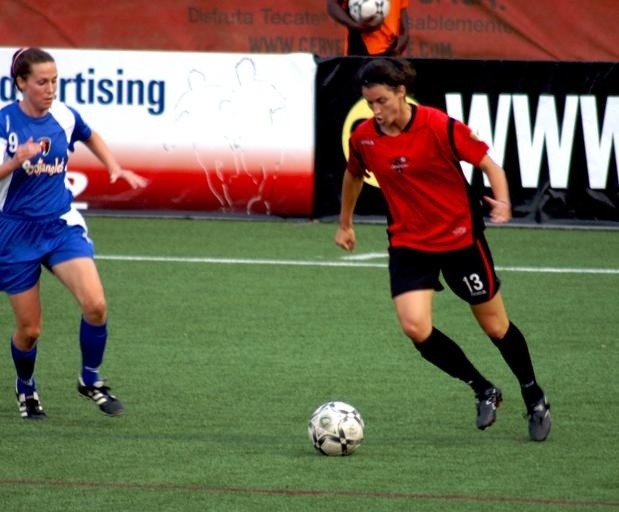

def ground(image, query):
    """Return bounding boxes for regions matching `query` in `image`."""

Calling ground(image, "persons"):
[335,58,551,440]
[0,46,151,418]
[328,0,412,55]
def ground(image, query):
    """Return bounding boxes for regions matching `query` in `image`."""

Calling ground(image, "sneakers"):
[78,375,125,417]
[524,389,551,442]
[16,378,48,421]
[475,380,502,430]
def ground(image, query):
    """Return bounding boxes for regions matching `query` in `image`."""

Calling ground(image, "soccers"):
[349,0,391,23]
[308,401,364,456]
